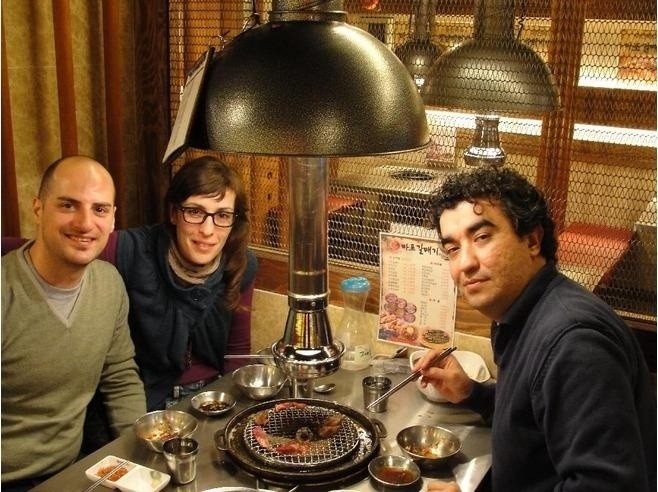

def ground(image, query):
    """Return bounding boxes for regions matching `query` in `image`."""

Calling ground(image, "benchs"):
[269,195,366,257]
[555,221,637,294]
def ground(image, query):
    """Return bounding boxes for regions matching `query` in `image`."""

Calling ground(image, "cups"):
[164,436,199,484]
[290,379,315,399]
[362,376,391,412]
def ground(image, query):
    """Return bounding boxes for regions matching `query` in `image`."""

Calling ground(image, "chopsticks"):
[221,352,275,361]
[365,348,457,408]
[84,458,129,490]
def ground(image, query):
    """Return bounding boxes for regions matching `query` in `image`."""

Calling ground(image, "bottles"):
[335,277,372,371]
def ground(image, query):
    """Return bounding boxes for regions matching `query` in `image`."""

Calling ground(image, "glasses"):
[173,203,239,228]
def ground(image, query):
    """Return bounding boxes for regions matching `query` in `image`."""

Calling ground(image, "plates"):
[191,391,237,415]
[421,329,451,347]
[84,454,171,492]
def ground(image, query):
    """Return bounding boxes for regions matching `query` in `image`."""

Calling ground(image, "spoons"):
[281,383,338,393]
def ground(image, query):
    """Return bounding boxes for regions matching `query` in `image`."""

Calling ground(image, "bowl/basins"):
[256,347,276,365]
[409,346,491,403]
[366,425,463,487]
[133,410,199,454]
[230,364,289,402]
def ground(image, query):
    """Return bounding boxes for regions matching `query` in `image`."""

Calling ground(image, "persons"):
[1,153,150,492]
[0,157,261,412]
[409,163,655,491]
